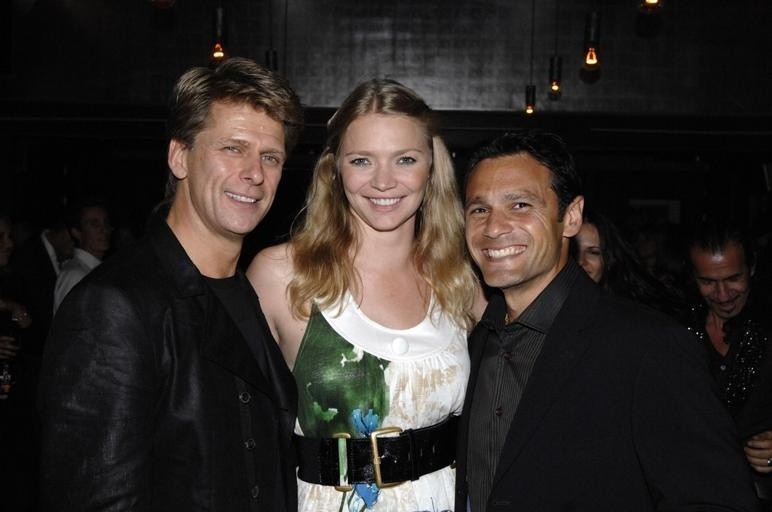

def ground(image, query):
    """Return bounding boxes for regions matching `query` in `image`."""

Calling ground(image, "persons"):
[244,75,489,512]
[452,127,760,511]
[52,196,112,318]
[0,214,33,360]
[24,55,306,512]
[574,209,644,298]
[680,209,772,478]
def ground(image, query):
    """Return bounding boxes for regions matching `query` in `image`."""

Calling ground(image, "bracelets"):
[11,304,29,321]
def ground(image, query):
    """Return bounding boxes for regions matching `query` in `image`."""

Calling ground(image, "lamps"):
[208,1,282,72]
[521,1,600,115]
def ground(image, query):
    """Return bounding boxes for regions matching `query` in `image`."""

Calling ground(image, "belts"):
[292,413,458,490]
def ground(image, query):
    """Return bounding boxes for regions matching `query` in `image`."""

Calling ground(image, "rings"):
[767,457,772,466]
